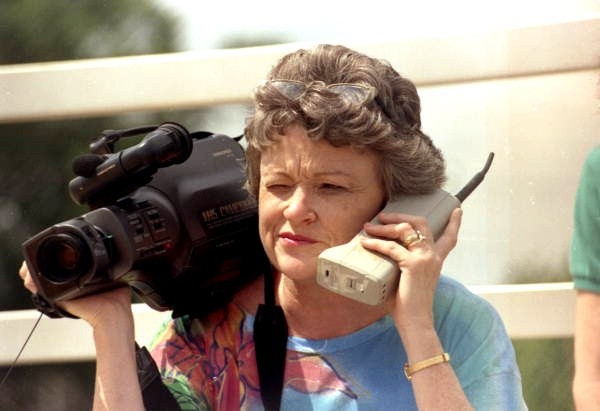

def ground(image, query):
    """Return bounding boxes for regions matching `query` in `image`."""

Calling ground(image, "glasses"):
[266,80,391,127]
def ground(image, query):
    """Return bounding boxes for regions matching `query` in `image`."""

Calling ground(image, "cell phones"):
[313,151,505,305]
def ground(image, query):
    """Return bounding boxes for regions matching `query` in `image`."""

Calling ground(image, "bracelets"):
[402,352,449,378]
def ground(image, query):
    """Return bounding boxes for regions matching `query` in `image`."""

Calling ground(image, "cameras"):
[20,120,265,319]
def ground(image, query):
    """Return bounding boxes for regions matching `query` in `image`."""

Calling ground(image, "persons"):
[571,144,600,411]
[20,44,529,411]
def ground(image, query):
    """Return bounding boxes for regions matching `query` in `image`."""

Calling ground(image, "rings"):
[404,231,425,248]
